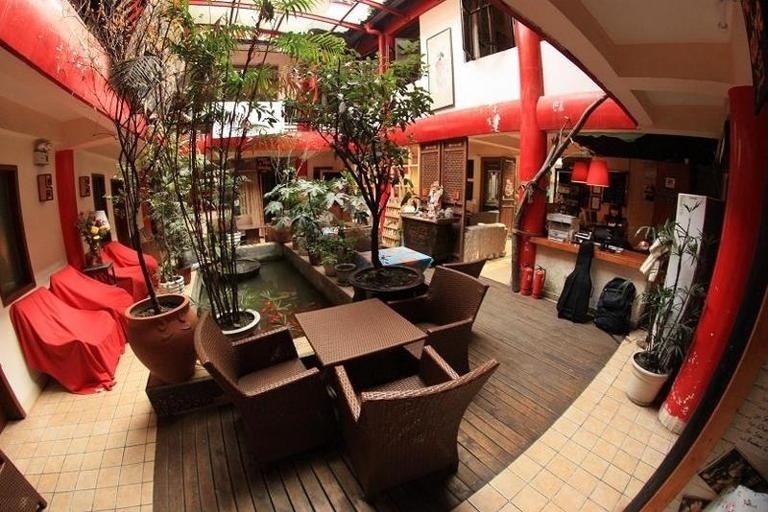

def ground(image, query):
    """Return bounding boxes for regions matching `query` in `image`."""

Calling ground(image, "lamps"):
[584,137,611,189]
[568,144,591,186]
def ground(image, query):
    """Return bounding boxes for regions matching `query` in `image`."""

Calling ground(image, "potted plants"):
[56,1,202,387]
[259,16,439,305]
[150,0,331,350]
[622,213,723,411]
[255,125,371,290]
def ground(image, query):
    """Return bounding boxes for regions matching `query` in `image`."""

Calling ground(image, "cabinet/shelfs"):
[476,154,517,238]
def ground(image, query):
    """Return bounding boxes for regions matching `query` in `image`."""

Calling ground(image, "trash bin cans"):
[0,450,47,512]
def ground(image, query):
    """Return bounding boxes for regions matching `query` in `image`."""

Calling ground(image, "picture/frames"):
[591,185,602,195]
[590,195,601,211]
[423,25,456,116]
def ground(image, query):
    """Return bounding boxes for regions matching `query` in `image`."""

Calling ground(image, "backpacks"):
[593,277,636,345]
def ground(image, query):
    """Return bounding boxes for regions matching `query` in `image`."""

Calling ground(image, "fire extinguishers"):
[520,263,546,298]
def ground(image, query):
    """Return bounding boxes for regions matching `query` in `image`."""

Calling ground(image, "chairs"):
[186,308,326,446]
[9,236,157,400]
[291,295,431,370]
[327,343,500,499]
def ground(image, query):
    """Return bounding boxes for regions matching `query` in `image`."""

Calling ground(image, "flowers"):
[71,208,108,266]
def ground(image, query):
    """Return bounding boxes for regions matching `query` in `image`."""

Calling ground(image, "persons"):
[427,181,443,209]
[601,204,633,251]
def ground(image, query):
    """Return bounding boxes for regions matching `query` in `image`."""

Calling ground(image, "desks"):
[386,261,491,373]
